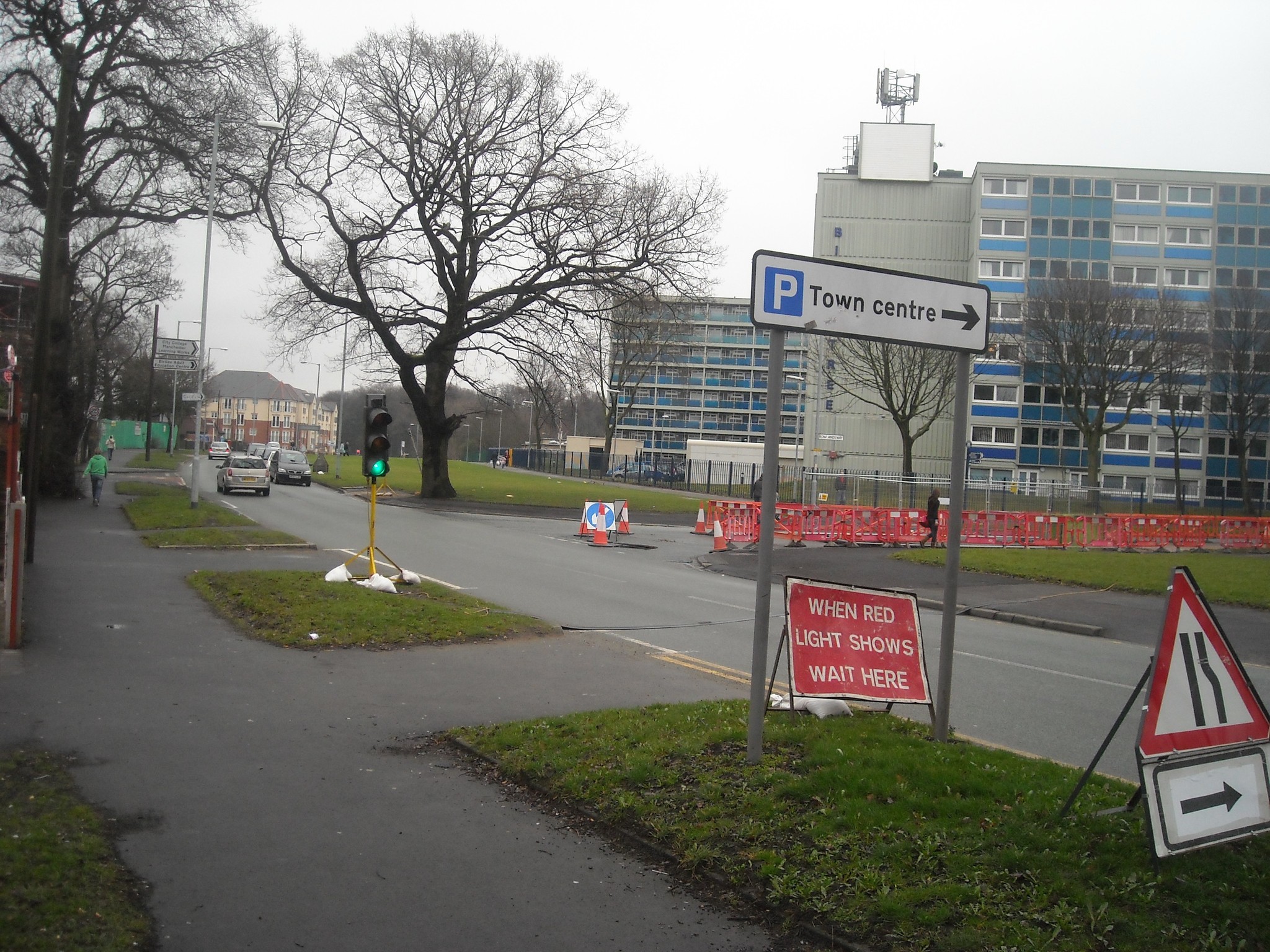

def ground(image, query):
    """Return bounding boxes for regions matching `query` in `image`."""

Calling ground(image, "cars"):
[243,442,283,472]
[215,454,271,496]
[607,459,686,483]
[269,448,311,488]
[208,441,234,460]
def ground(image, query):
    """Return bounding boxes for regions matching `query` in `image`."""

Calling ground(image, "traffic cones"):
[690,501,708,535]
[709,510,733,553]
[573,500,634,547]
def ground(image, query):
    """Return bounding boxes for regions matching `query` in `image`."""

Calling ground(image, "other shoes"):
[94,499,99,506]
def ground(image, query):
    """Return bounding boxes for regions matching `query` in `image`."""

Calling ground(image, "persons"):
[340,442,349,457]
[750,474,763,523]
[834,472,847,505]
[82,447,108,506]
[491,450,510,469]
[290,440,295,450]
[105,436,116,461]
[918,489,940,549]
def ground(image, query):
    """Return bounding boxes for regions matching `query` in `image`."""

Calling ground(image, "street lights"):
[610,389,620,478]
[660,415,670,465]
[202,346,229,452]
[784,374,806,503]
[0,282,26,356]
[522,401,533,470]
[300,361,321,400]
[190,116,287,506]
[462,409,503,462]
[410,423,419,451]
[170,320,202,458]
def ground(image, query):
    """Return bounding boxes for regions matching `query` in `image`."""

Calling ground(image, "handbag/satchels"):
[920,516,929,528]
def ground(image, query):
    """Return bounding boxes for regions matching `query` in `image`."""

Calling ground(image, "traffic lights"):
[362,393,393,477]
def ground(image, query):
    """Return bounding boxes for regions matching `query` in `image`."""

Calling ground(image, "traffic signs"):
[155,337,199,357]
[182,392,206,401]
[750,250,992,355]
[152,357,197,371]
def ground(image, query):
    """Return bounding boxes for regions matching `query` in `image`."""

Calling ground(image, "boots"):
[919,536,929,548]
[931,542,935,549]
